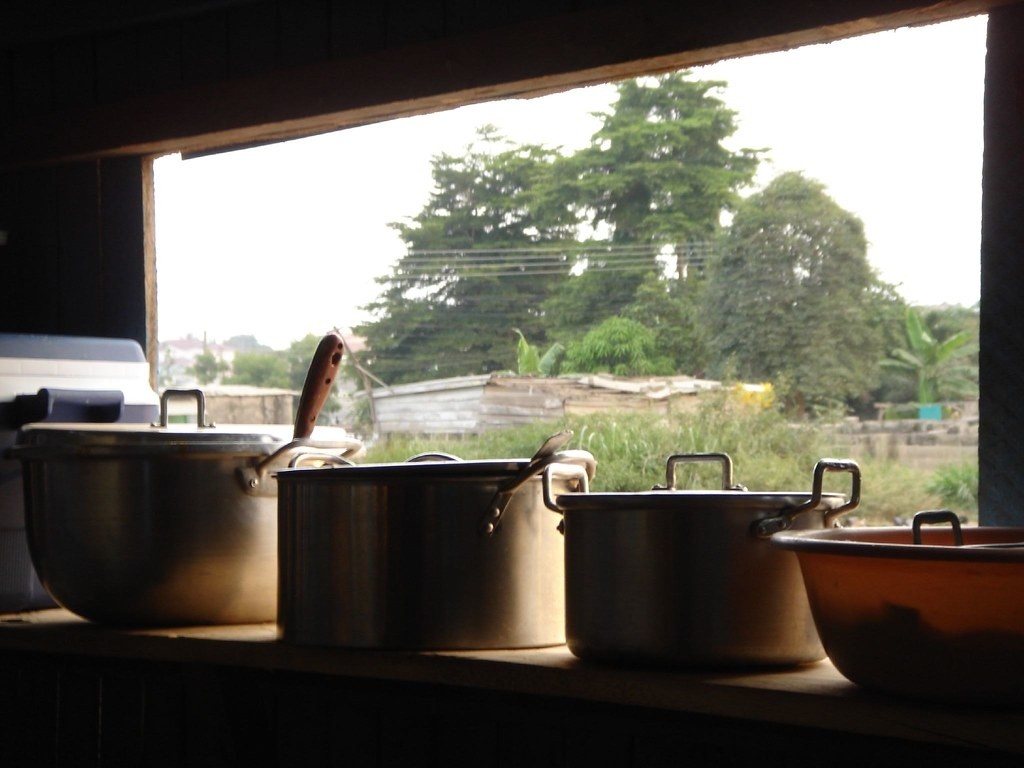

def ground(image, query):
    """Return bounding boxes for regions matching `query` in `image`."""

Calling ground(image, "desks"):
[0,607,1024,768]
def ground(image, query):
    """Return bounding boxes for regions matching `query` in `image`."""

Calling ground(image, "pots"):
[554,452,863,670]
[17,390,363,628]
[765,505,1024,710]
[274,431,600,652]
